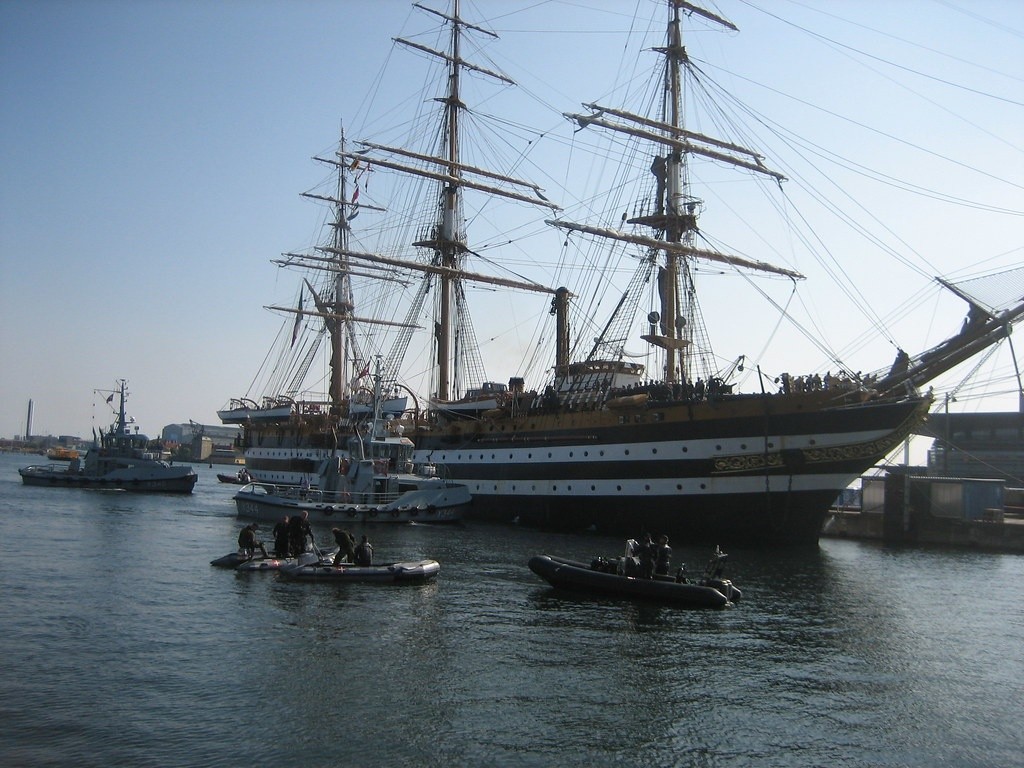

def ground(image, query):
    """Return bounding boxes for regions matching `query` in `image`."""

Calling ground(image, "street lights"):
[945,392,957,476]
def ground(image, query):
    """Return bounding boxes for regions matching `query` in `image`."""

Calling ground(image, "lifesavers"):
[347,507,357,518]
[411,507,418,517]
[428,505,435,514]
[343,493,351,504]
[391,508,400,519]
[369,508,378,518]
[375,461,385,475]
[324,506,334,516]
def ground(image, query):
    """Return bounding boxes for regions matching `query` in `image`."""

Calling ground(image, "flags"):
[356,361,372,382]
[289,281,304,352]
[106,394,114,404]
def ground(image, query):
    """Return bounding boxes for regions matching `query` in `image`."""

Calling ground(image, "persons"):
[273,511,316,558]
[926,384,935,399]
[631,533,673,581]
[621,374,716,401]
[592,379,600,393]
[332,527,373,568]
[601,378,609,394]
[238,522,270,559]
[788,370,877,392]
[237,432,241,442]
[237,468,254,479]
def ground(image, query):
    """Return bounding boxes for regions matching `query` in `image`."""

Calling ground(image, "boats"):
[527,539,743,611]
[216,0,1024,555]
[47,447,81,461]
[232,352,473,524]
[18,378,198,494]
[216,407,293,425]
[210,546,440,587]
[216,472,256,484]
